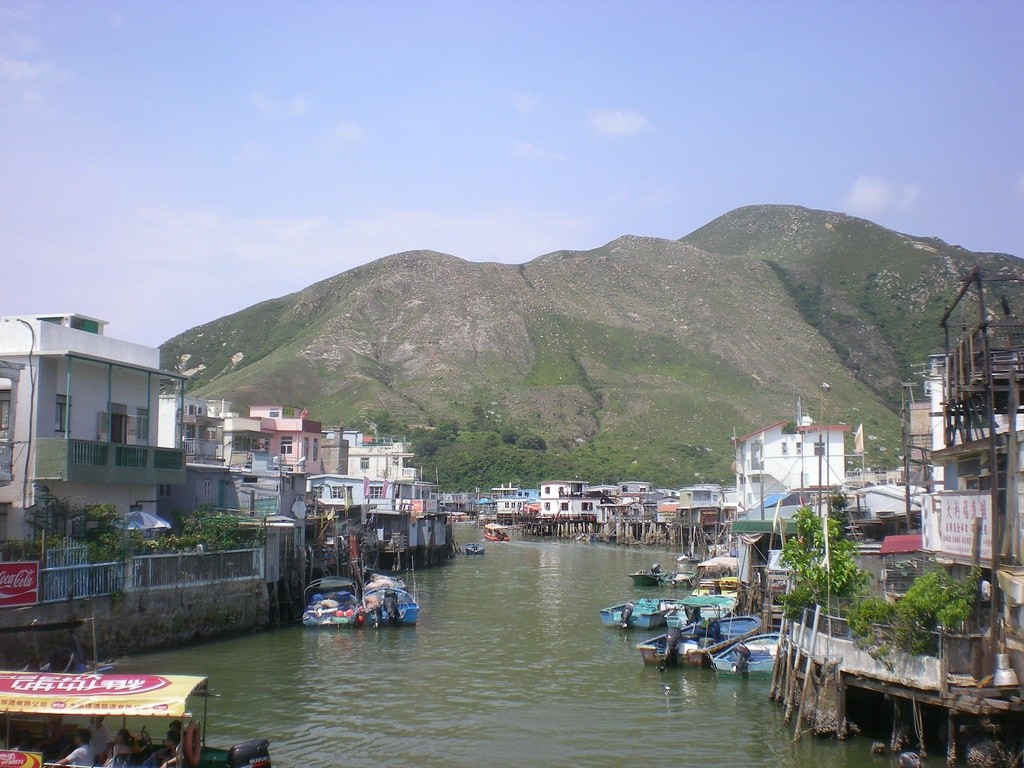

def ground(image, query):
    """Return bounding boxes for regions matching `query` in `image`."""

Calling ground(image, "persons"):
[0,713,186,768]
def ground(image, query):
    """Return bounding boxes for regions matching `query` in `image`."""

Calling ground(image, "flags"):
[380,480,389,498]
[364,477,370,497]
[854,424,863,453]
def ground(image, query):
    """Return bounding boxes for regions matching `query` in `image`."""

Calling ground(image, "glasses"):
[72,734,81,738]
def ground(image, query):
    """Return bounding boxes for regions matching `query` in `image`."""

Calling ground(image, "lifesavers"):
[185,720,200,768]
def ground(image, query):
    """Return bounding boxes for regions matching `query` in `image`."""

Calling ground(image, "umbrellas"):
[109,510,172,531]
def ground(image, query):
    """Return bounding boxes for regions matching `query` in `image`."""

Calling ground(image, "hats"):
[118,735,134,747]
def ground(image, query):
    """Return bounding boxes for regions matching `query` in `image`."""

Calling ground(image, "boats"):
[665,595,739,630]
[599,598,681,629]
[301,573,366,627]
[690,576,740,605]
[635,615,763,667]
[671,552,702,564]
[463,542,486,555]
[712,632,786,672]
[664,571,696,588]
[1,667,272,768]
[364,575,407,590]
[362,588,420,626]
[0,618,118,674]
[446,512,476,528]
[480,524,510,541]
[626,563,668,587]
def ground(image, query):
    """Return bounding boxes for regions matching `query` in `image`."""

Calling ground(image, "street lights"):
[818,382,832,517]
